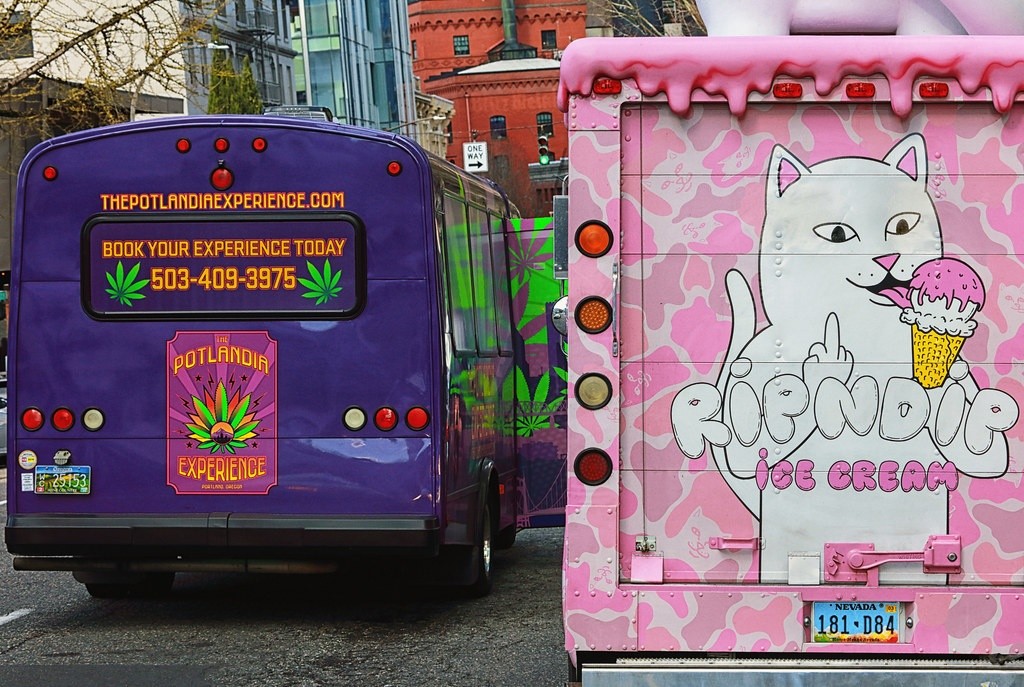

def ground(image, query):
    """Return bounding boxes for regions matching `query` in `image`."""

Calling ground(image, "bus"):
[5,107,524,601]
[549,0,1024,687]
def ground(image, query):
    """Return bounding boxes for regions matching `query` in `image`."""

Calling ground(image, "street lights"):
[129,45,231,124]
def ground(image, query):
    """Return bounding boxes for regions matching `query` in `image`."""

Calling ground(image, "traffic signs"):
[463,141,489,174]
